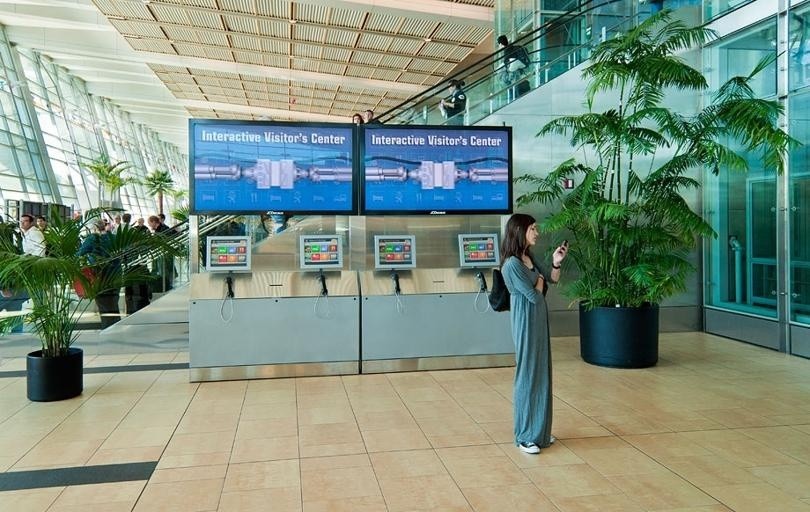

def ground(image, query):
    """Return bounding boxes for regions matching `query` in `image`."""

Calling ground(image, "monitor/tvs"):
[299,235,343,268]
[206,236,252,271]
[360,124,513,216]
[189,118,358,216]
[374,234,417,269]
[458,233,501,267]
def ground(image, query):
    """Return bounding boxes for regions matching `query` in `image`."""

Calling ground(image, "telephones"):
[391,273,400,294]
[476,271,487,291]
[226,277,234,297]
[318,275,328,295]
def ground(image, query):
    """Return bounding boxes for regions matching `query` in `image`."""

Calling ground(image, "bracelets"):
[552,261,563,270]
[538,275,545,281]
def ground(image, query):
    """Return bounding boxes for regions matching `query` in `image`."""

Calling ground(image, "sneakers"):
[520,435,555,454]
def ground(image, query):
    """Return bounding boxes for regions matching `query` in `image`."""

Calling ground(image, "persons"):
[200,214,294,269]
[499,212,571,454]
[441,79,468,126]
[1,214,187,333]
[363,110,382,125]
[496,35,532,101]
[351,113,365,125]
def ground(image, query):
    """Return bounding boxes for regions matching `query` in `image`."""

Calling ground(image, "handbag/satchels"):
[490,270,509,312]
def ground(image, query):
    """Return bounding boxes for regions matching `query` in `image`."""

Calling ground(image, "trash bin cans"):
[125,264,152,314]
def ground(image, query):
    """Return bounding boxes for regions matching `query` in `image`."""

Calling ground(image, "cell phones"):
[559,240,568,253]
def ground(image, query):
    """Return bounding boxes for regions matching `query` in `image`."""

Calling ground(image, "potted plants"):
[1,205,184,404]
[513,12,806,372]
